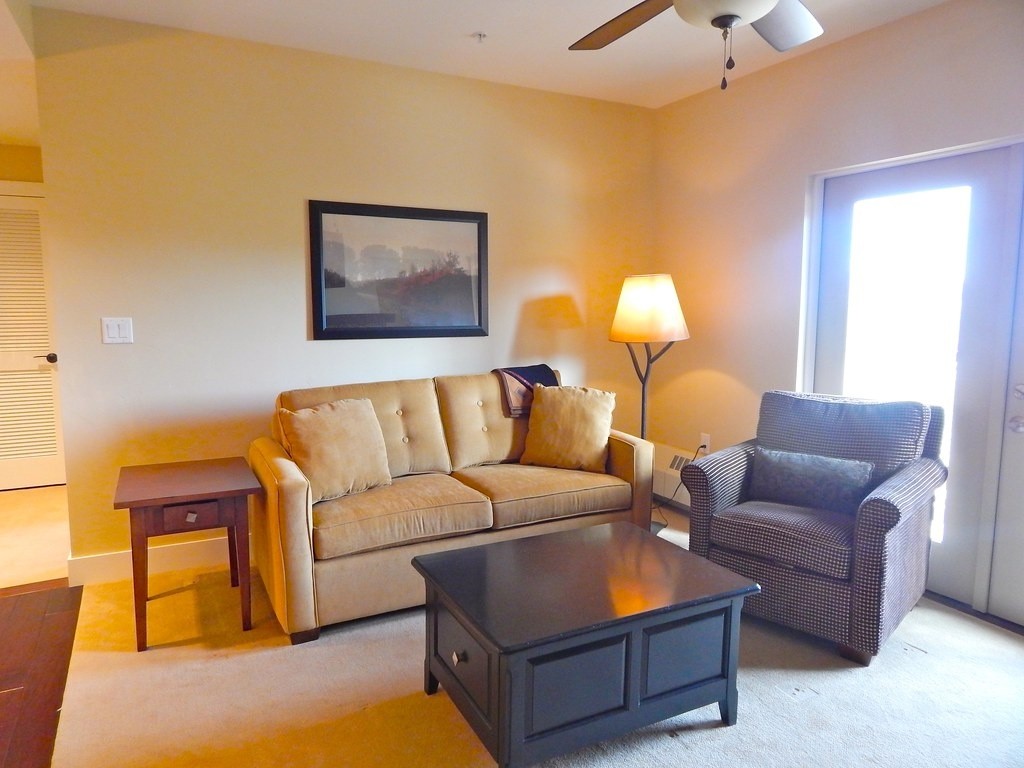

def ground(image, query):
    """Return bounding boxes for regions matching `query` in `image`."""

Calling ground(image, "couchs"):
[249,364,654,645]
[680,389,949,667]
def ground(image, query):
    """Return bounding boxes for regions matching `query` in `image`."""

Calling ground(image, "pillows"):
[277,397,393,505]
[750,445,876,516]
[519,383,617,474]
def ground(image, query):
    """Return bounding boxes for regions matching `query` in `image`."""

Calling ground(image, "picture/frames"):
[308,199,489,341]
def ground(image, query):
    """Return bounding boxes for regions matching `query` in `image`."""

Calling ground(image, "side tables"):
[113,456,263,653]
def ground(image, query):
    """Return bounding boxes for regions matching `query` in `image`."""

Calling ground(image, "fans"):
[568,0,825,52]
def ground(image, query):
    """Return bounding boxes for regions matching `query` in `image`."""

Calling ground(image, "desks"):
[409,523,762,768]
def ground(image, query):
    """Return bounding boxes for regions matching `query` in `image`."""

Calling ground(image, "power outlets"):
[700,433,710,455]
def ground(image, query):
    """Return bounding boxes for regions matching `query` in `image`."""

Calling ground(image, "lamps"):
[610,274,689,535]
[673,0,779,90]
